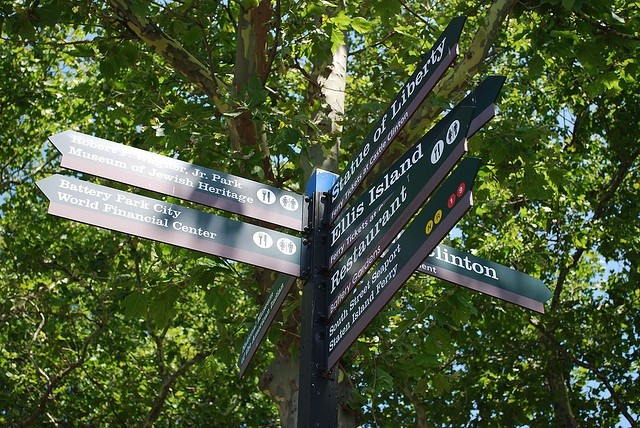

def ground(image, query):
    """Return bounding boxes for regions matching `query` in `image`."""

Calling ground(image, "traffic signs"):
[237,272,298,380]
[321,16,467,230]
[34,173,312,280]
[379,229,554,315]
[312,157,482,377]
[319,76,507,277]
[47,130,310,232]
[315,106,477,324]
